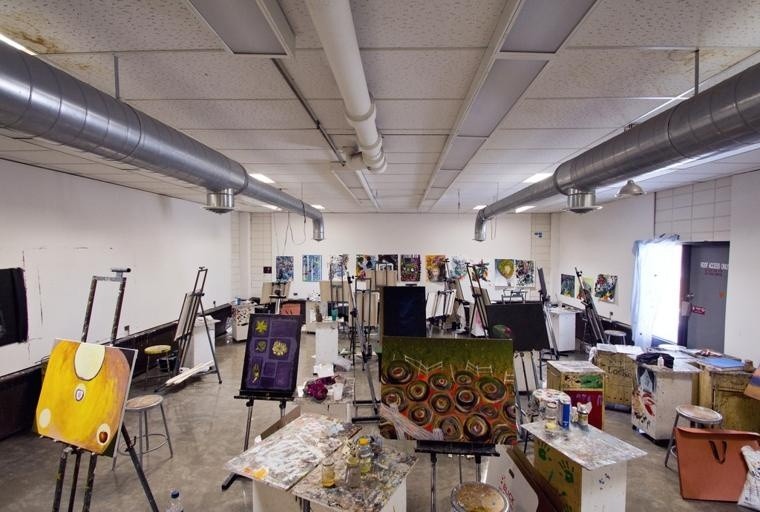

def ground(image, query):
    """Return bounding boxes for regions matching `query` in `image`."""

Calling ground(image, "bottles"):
[657,354,664,368]
[541,397,591,433]
[743,359,754,372]
[318,435,373,490]
[165,489,184,512]
[316,309,322,321]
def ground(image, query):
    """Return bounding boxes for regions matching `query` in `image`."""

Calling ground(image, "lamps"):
[613,179,645,198]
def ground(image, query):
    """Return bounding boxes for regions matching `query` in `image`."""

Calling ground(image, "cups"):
[332,383,344,401]
[236,297,240,305]
[331,308,338,321]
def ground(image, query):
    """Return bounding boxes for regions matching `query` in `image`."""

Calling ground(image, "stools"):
[605,330,627,345]
[111,394,173,472]
[450,481,509,512]
[144,345,172,391]
[664,404,724,468]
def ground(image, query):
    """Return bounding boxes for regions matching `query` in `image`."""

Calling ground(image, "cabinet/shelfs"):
[221,412,363,512]
[520,419,649,512]
[545,307,583,353]
[172,319,222,377]
[547,344,760,444]
[314,321,339,371]
[231,304,256,343]
[289,430,420,512]
